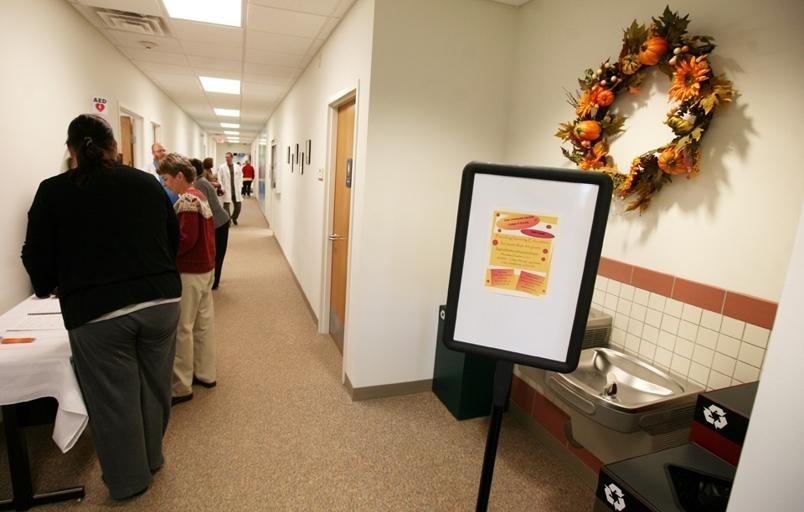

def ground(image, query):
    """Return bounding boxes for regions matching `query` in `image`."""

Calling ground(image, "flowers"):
[555,2,743,217]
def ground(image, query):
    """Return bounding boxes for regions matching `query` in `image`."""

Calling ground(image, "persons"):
[21,113,182,499]
[151,140,257,407]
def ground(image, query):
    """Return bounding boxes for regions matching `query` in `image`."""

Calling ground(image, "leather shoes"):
[230,216,238,225]
[169,394,193,407]
[191,374,217,387]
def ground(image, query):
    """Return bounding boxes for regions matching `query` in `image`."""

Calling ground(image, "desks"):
[0,292,92,511]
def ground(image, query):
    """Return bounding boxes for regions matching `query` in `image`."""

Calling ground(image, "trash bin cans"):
[432,305,514,421]
[592,380,759,512]
